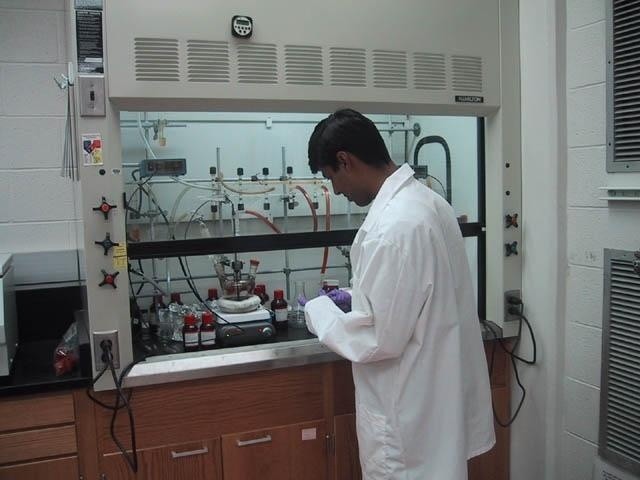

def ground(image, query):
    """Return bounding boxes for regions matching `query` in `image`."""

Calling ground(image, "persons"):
[297,108,498,480]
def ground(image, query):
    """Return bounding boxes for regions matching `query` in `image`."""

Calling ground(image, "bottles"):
[208,166,319,214]
[148,258,338,351]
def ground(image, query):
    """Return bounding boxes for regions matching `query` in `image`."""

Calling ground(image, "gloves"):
[296,287,353,315]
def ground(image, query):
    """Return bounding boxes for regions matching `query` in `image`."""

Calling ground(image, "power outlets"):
[503,289,522,322]
[92,329,121,372]
